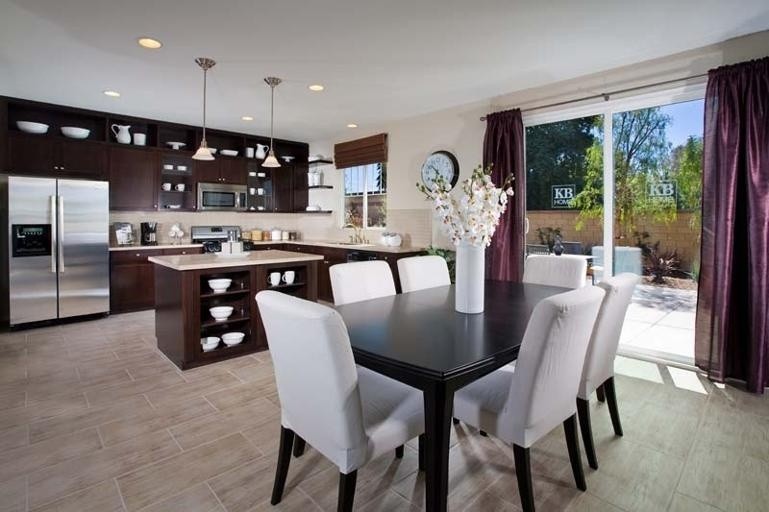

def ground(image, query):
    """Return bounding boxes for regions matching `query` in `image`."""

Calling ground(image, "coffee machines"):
[140,222,158,246]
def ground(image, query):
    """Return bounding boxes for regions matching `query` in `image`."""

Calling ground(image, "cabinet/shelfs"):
[164,248,204,255]
[254,244,319,257]
[1,94,107,180]
[159,121,196,212]
[109,112,158,212]
[309,157,333,215]
[274,138,309,213]
[197,126,245,184]
[245,135,273,213]
[110,249,163,315]
[318,248,427,305]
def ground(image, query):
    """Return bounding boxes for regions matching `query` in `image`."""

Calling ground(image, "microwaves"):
[197,182,248,211]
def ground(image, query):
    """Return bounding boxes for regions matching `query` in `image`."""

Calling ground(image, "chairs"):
[522,255,588,287]
[395,257,460,426]
[576,271,642,470]
[328,257,404,462]
[453,287,605,512]
[254,290,426,512]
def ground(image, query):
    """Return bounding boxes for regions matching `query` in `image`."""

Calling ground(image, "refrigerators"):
[7,176,112,329]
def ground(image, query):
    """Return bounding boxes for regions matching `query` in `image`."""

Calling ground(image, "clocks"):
[420,150,459,194]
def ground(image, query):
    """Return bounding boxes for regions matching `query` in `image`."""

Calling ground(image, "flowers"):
[415,164,517,246]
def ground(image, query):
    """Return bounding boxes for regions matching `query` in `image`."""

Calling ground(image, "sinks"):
[334,241,377,249]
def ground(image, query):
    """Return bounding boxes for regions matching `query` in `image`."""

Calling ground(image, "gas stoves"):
[192,237,254,252]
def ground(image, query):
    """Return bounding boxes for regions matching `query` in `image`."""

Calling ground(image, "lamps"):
[259,72,283,169]
[191,52,218,163]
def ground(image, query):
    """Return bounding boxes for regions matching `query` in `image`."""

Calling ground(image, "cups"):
[258,188,265,195]
[282,271,296,285]
[175,183,186,191]
[268,272,281,286]
[249,187,256,195]
[246,147,254,158]
[221,241,244,254]
[132,133,148,145]
[160,182,173,191]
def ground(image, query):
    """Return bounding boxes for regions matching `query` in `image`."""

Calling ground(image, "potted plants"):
[427,246,456,285]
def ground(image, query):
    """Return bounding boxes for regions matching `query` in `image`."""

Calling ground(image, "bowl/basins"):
[61,126,92,139]
[209,306,234,321]
[208,278,232,292]
[17,120,50,133]
[200,337,220,352]
[221,332,245,347]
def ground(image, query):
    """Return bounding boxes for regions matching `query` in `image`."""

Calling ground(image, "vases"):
[452,243,488,315]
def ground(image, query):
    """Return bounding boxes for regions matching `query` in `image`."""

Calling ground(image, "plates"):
[215,252,250,256]
[219,150,239,157]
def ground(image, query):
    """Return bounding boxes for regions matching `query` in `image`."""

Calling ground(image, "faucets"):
[342,222,358,244]
